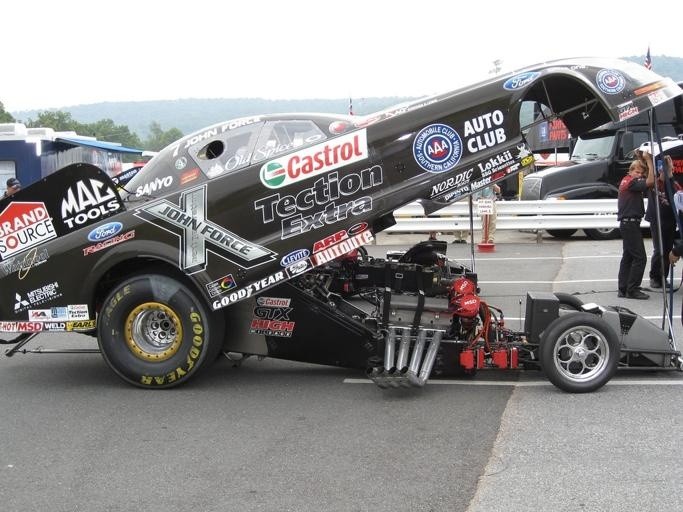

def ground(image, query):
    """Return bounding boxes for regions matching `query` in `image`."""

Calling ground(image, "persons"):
[671,173,682,231]
[668,208,682,326]
[645,152,675,288]
[452,197,469,244]
[617,148,654,300]
[0,178,21,200]
[475,184,500,245]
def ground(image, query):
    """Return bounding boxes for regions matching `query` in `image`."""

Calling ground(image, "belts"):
[620,217,641,222]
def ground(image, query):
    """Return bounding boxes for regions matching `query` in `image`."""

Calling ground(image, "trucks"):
[518,81,683,239]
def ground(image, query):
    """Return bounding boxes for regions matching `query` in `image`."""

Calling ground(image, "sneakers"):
[616,278,675,300]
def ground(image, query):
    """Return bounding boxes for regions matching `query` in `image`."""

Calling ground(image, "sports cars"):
[0,58,683,393]
